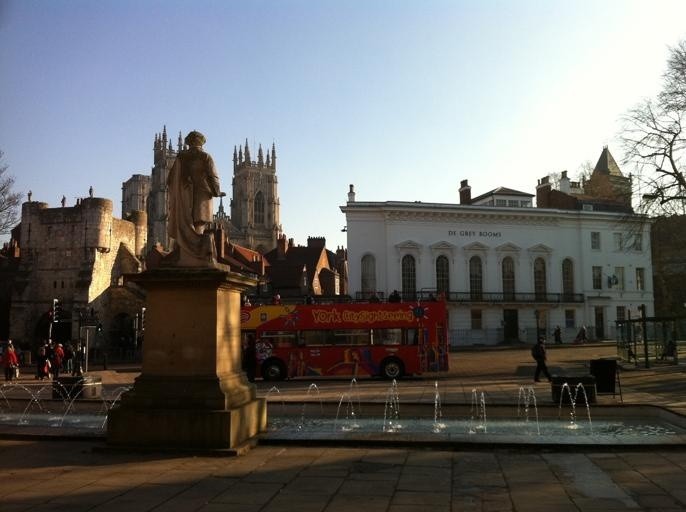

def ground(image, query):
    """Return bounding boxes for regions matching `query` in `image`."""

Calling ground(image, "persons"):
[553,326,563,345]
[35,339,86,380]
[3,344,18,381]
[243,334,256,384]
[162,131,221,268]
[6,340,14,352]
[531,338,555,383]
[576,326,587,342]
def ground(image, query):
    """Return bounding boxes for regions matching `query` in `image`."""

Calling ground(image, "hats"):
[58,344,63,347]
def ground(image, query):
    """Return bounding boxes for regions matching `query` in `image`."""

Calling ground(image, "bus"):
[241,287,451,383]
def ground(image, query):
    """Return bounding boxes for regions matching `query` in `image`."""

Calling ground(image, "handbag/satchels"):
[50,366,57,374]
[10,365,16,369]
[40,363,48,374]
[15,369,19,378]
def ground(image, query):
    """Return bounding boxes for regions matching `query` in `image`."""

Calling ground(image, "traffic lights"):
[46,297,62,324]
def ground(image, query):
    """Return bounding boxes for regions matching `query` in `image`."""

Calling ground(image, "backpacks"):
[532,347,539,360]
